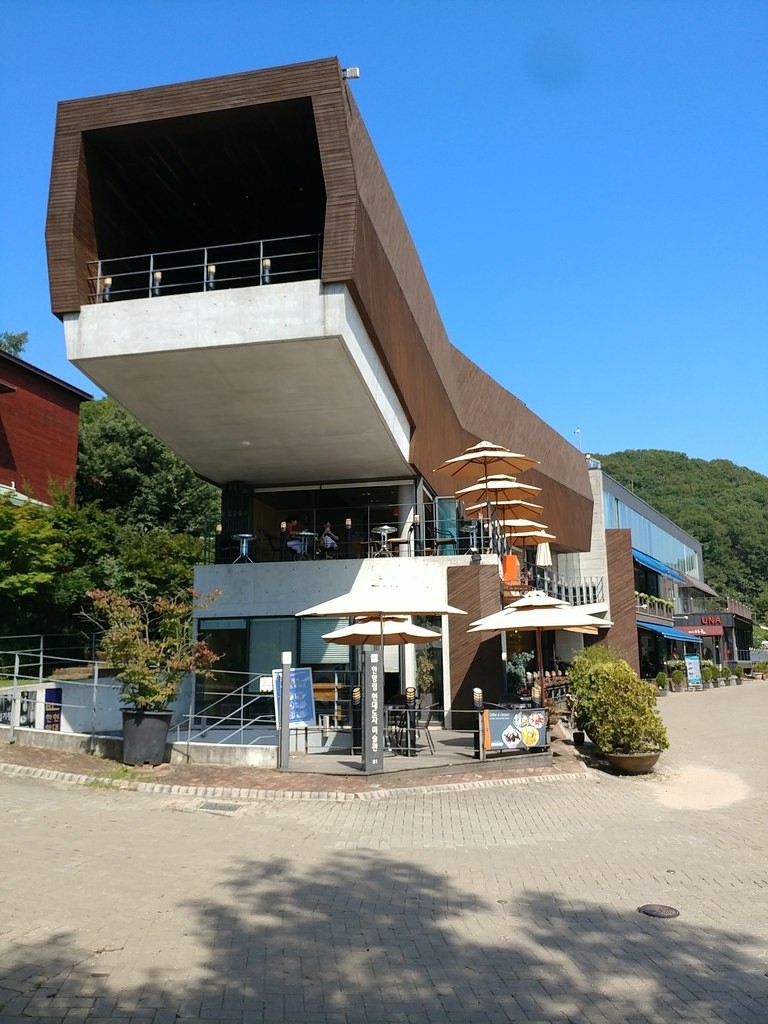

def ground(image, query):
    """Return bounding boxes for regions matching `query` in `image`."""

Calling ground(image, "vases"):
[120,708,174,768]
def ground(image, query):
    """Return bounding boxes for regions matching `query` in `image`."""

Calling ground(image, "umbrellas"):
[499,531,556,571]
[294,576,468,671]
[467,590,615,708]
[432,440,541,502]
[320,614,442,645]
[468,607,598,635]
[465,500,544,538]
[454,474,542,527]
[484,518,549,555]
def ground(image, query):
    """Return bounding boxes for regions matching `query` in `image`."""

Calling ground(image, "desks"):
[370,527,397,557]
[460,525,479,556]
[231,533,257,564]
[294,532,319,560]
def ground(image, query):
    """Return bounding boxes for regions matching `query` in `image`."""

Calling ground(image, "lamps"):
[154,272,162,281]
[103,278,113,287]
[281,522,287,533]
[262,258,272,269]
[207,265,216,276]
[346,519,352,529]
[214,522,223,536]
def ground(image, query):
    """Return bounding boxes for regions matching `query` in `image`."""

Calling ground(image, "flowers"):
[85,587,228,711]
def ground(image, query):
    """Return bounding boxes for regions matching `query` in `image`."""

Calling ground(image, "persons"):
[285,517,312,561]
[553,656,562,671]
[320,521,340,559]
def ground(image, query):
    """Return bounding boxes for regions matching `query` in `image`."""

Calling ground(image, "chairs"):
[428,526,457,555]
[320,544,341,560]
[398,697,424,737]
[263,530,294,561]
[400,702,440,755]
[388,522,417,557]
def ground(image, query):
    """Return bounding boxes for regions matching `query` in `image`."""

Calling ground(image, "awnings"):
[678,571,719,598]
[632,547,686,584]
[637,621,703,642]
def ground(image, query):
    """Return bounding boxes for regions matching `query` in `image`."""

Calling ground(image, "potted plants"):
[711,665,720,687]
[672,670,684,691]
[572,661,672,774]
[655,672,668,697]
[701,668,715,690]
[634,591,674,612]
[721,666,731,686]
[735,665,744,684]
[752,663,764,680]
